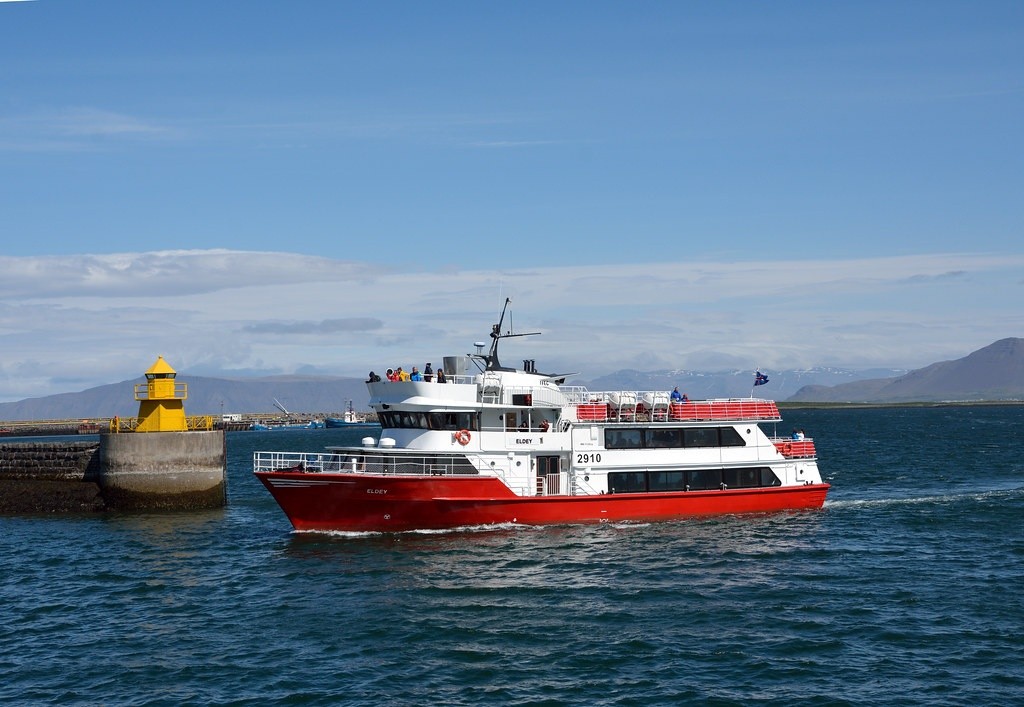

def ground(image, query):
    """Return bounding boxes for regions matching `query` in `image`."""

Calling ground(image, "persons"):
[604,427,782,493]
[539,419,550,432]
[410,367,421,382]
[424,363,433,382]
[385,366,411,382]
[437,368,447,383]
[671,387,690,404]
[365,371,381,383]
[518,421,529,432]
[792,427,805,442]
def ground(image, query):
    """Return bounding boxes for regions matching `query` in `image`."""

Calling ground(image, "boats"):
[252,296,832,543]
[77,420,104,434]
[252,418,322,430]
[323,398,382,428]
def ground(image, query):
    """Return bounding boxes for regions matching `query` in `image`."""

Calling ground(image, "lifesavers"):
[458,429,471,445]
[782,443,793,456]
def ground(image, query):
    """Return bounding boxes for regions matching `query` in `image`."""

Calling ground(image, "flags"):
[754,370,769,386]
